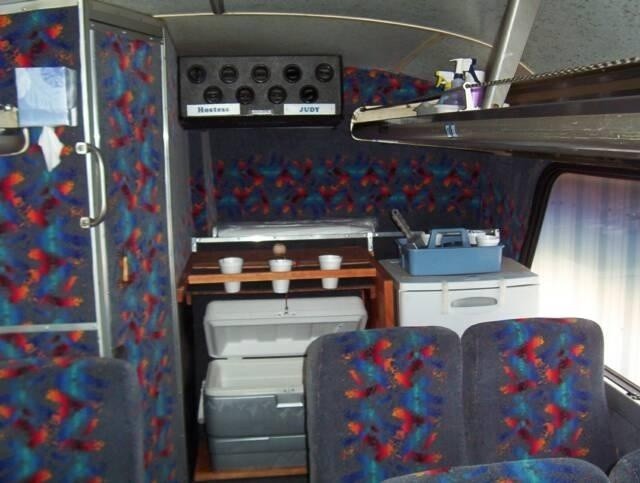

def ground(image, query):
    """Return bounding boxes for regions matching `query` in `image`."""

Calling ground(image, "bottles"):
[464,70,486,107]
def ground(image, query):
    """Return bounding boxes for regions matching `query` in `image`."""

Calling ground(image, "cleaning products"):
[449,57,481,105]
[435,71,454,91]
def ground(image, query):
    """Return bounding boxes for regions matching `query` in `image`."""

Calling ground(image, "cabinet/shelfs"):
[380,258,542,337]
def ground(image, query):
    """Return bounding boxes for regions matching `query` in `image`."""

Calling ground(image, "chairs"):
[384,459,609,483]
[303,327,466,483]
[609,450,640,483]
[460,317,616,476]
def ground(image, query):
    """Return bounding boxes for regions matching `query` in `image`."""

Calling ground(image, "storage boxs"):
[194,294,370,472]
[395,227,505,276]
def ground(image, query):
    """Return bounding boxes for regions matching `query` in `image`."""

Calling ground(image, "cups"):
[269,259,292,294]
[318,254,342,290]
[423,226,501,246]
[219,257,244,294]
